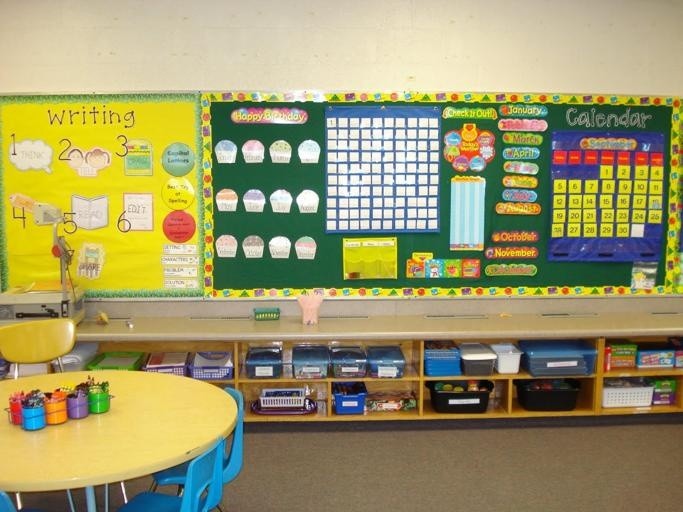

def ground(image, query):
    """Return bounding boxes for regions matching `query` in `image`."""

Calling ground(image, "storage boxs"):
[424,342,599,378]
[243,343,407,380]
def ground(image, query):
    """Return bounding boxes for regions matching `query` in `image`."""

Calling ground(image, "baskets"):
[87,351,234,381]
[259,388,305,408]
[603,386,654,408]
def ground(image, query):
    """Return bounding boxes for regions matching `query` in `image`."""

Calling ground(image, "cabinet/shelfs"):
[77,314,682,421]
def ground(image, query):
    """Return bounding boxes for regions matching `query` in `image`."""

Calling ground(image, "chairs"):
[0,317,76,511]
[118,386,244,512]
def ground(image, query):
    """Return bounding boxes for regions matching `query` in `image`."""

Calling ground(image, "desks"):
[0,368,239,511]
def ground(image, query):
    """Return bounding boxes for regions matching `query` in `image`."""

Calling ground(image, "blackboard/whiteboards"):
[205,92,683,301]
[0,90,203,299]
